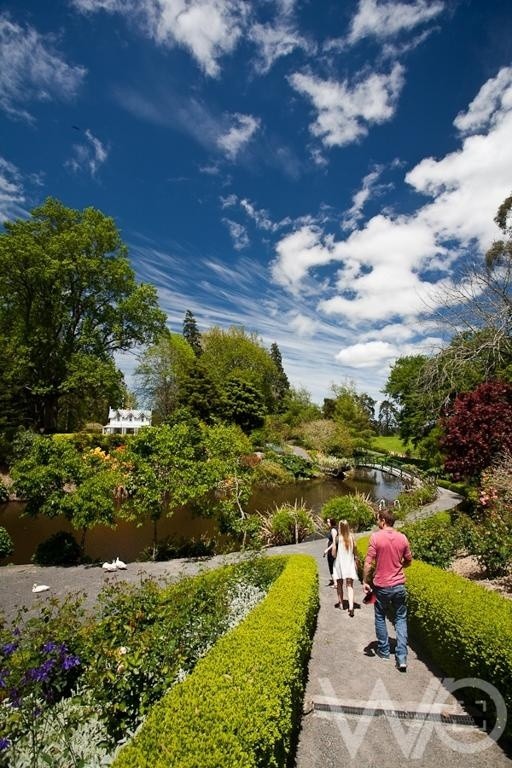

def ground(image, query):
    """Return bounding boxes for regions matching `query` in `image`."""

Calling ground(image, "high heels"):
[335,601,343,609]
[349,610,355,617]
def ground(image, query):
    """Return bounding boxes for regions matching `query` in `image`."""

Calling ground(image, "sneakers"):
[376,646,390,658]
[395,655,407,671]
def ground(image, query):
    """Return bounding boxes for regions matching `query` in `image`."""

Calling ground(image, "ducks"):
[101,556,127,572]
[31,582,51,593]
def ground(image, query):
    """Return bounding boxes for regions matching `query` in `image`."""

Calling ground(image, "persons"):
[322,515,340,589]
[332,519,359,617]
[361,508,413,670]
